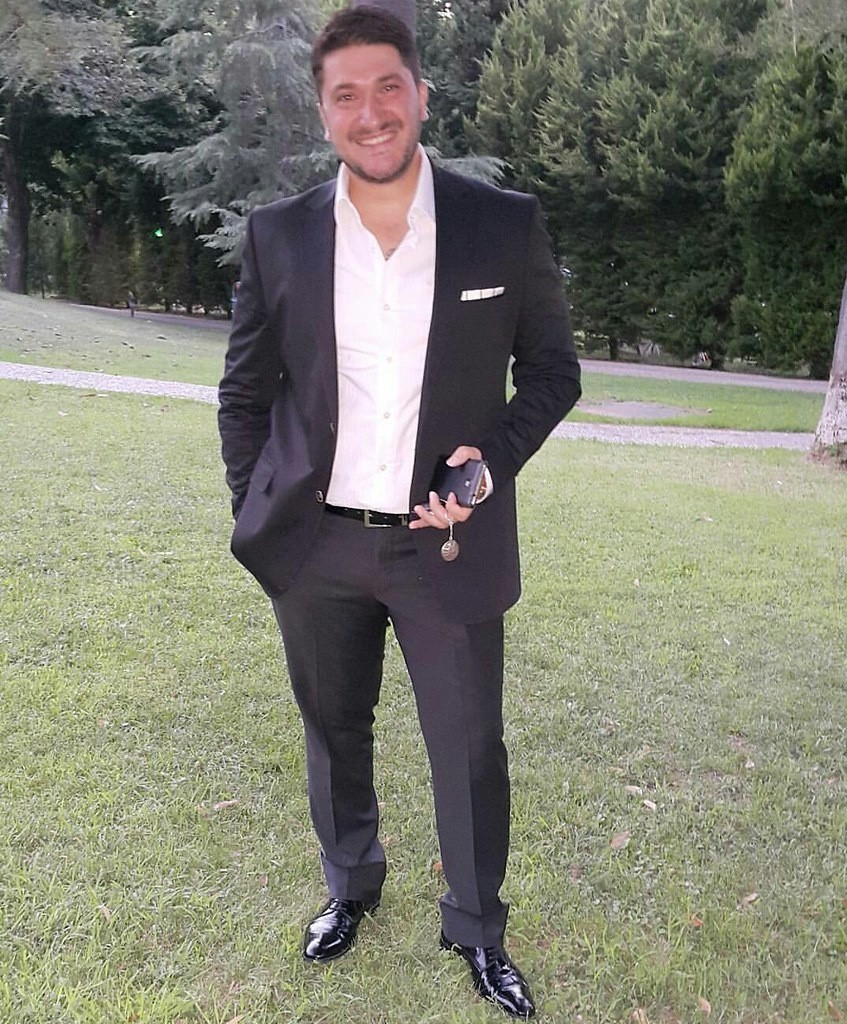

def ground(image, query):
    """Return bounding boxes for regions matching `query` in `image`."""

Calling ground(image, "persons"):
[218,5,583,1017]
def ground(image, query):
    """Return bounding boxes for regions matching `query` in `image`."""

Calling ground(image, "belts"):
[325,504,409,530]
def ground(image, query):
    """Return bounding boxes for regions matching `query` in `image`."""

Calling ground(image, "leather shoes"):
[440,927,536,1019]
[302,894,381,963]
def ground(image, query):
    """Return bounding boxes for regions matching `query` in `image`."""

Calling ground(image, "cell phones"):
[429,454,486,509]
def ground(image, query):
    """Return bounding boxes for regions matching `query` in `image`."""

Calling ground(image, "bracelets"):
[477,471,486,499]
